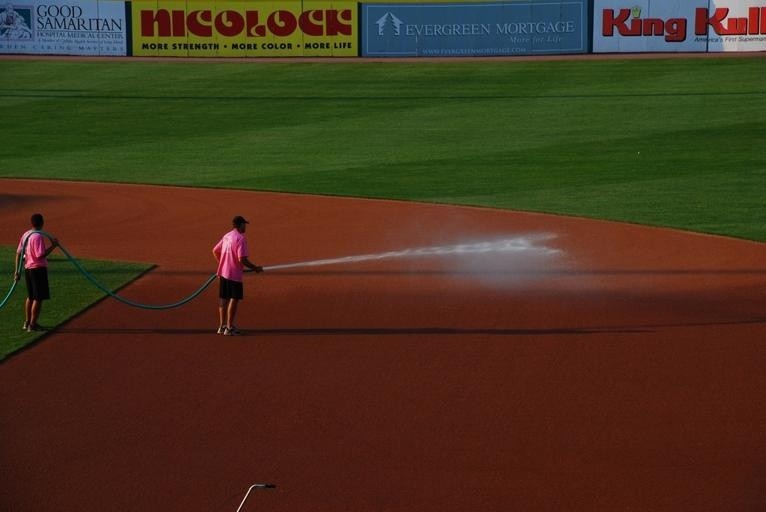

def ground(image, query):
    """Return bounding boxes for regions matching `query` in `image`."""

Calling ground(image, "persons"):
[213,216,258,335]
[14,213,58,332]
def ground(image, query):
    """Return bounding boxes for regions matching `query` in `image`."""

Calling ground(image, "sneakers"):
[22,321,46,333]
[217,324,227,334]
[223,327,245,337]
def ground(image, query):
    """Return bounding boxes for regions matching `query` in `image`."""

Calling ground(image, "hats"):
[232,216,250,225]
